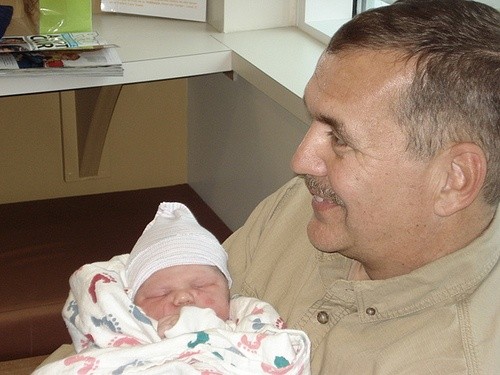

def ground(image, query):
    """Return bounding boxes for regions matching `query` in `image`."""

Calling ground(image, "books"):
[0,31,124,77]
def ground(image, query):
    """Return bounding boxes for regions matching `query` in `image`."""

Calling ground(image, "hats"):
[123,201,232,302]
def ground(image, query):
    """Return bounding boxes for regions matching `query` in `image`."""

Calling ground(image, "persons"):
[34,203,311,374]
[34,0,500,375]
